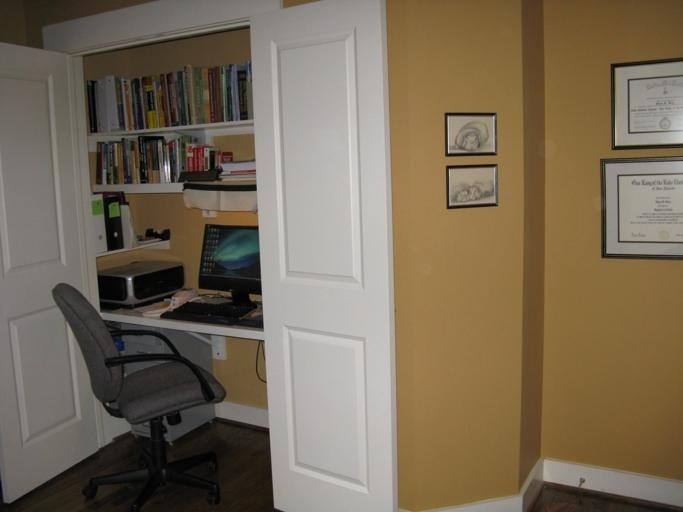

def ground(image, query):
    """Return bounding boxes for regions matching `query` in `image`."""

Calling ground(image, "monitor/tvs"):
[198,222,262,313]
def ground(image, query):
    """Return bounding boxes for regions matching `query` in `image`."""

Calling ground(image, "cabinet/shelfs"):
[81,27,267,342]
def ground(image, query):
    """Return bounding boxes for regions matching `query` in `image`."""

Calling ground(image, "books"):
[84,59,256,186]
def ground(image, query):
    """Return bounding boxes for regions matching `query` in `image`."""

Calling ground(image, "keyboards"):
[159,299,240,327]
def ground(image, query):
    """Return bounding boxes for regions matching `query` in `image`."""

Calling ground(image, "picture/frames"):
[445,163,498,208]
[598,156,683,261]
[608,57,682,148]
[444,110,498,156]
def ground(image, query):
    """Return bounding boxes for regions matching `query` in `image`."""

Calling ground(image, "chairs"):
[51,282,228,511]
